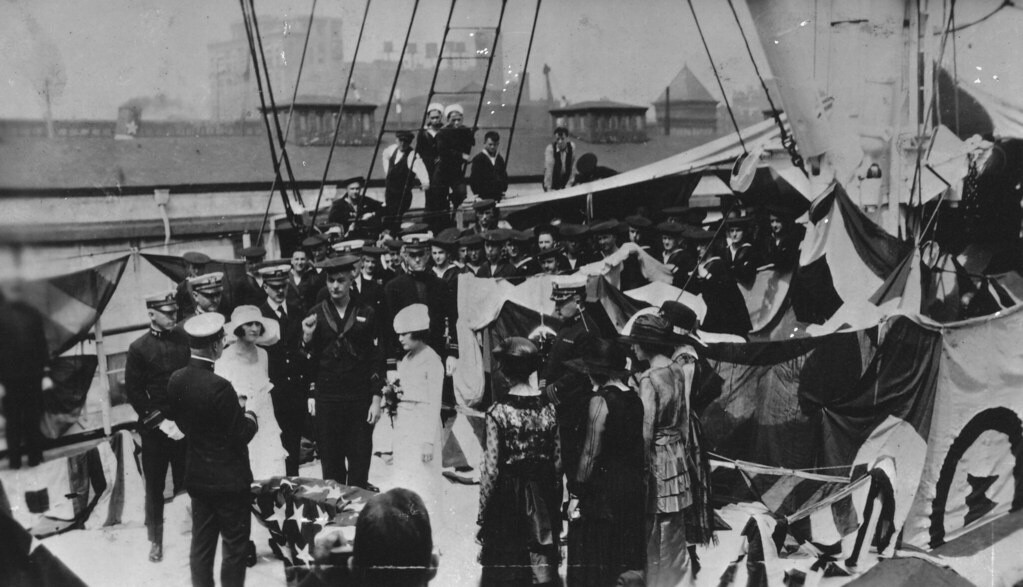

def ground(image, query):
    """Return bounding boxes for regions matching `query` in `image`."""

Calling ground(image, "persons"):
[383,130,430,209]
[167,312,269,587]
[541,127,578,193]
[368,303,445,554]
[124,198,792,587]
[0,275,50,470]
[469,131,509,200]
[297,255,388,493]
[416,102,476,208]
[572,152,619,186]
[327,176,384,235]
[473,335,566,587]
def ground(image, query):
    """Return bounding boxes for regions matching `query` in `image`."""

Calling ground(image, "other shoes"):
[366,482,379,492]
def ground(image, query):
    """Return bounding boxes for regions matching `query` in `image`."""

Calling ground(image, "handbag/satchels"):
[525,473,564,516]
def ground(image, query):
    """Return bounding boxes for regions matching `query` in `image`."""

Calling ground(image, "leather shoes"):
[149,544,163,564]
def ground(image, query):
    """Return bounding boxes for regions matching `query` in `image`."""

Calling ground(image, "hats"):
[546,272,588,302]
[256,264,292,280]
[222,305,281,347]
[146,289,180,313]
[575,152,599,177]
[186,272,226,295]
[336,177,366,188]
[240,247,267,261]
[395,131,415,140]
[444,104,465,121]
[391,304,432,335]
[302,197,791,256]
[313,255,363,273]
[182,250,210,267]
[618,300,712,347]
[562,338,637,380]
[182,313,228,348]
[491,335,546,375]
[426,103,443,114]
